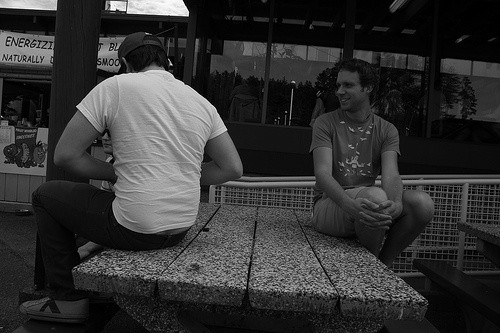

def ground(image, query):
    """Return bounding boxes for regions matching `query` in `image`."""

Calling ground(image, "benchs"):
[379,318,440,333]
[11,292,121,333]
[71,202,429,333]
[412,258,500,333]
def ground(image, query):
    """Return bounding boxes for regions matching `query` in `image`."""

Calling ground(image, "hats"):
[118,32,165,62]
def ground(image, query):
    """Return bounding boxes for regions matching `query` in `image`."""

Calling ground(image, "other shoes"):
[20,284,71,298]
[17,297,92,325]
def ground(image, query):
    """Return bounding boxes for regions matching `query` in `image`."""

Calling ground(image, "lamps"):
[388,0,408,14]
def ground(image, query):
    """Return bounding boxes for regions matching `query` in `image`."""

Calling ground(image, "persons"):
[77,128,116,259]
[310,59,435,268]
[19,32,242,323]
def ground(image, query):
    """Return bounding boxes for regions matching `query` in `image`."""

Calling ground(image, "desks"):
[457,222,500,269]
